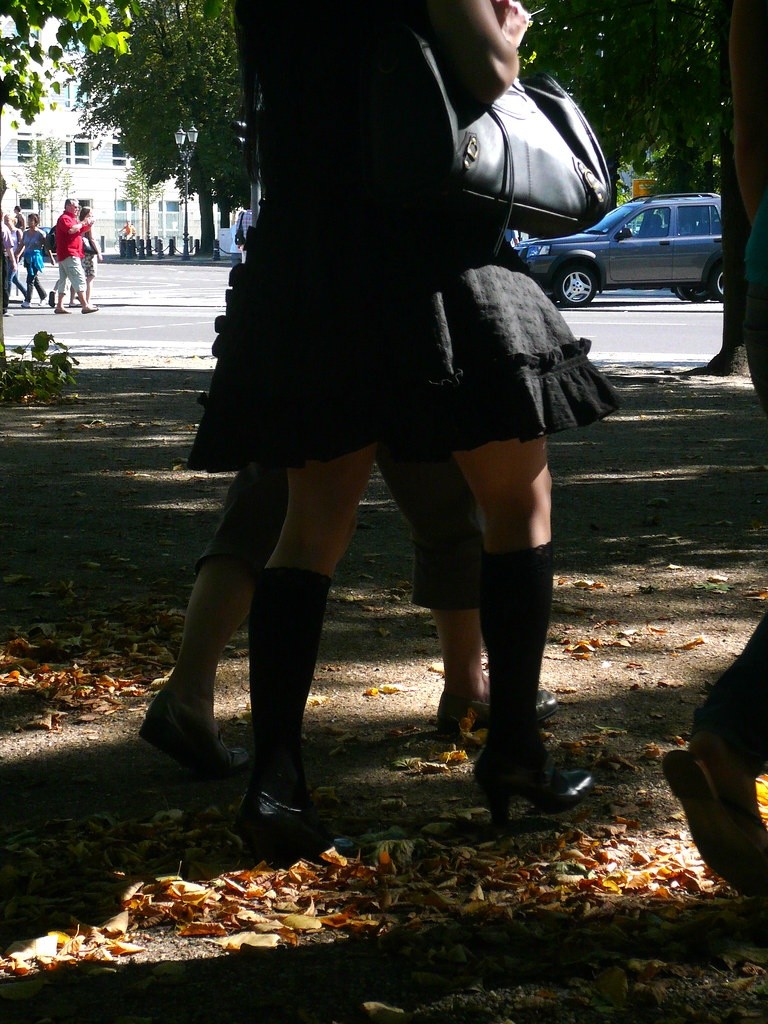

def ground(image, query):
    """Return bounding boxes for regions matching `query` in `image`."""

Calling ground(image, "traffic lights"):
[229,120,246,151]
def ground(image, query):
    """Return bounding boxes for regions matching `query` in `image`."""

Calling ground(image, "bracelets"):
[81,222,85,227]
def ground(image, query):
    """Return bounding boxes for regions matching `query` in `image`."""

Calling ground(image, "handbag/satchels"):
[82,236,101,254]
[234,213,245,245]
[398,23,615,239]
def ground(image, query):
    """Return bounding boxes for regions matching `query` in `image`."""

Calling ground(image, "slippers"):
[663,748,767,894]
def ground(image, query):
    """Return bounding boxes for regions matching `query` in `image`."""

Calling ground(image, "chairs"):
[645,215,662,237]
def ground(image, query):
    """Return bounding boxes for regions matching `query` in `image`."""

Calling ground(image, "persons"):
[119,219,136,240]
[0,198,104,317]
[139,0,596,870]
[663,0,768,908]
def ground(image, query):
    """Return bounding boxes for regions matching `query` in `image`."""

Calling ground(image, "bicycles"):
[114,233,133,252]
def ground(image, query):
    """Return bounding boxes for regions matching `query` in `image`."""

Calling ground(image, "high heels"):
[472,742,601,823]
[234,789,355,865]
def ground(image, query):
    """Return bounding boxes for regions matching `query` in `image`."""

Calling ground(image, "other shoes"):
[38,296,48,306]
[81,309,98,314]
[436,688,557,746]
[140,686,250,774]
[69,303,82,307]
[49,291,55,307]
[21,301,32,308]
[54,308,71,314]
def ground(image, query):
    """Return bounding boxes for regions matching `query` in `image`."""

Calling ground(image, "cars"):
[38,227,53,256]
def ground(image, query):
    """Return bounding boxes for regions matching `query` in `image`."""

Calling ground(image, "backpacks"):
[46,226,60,253]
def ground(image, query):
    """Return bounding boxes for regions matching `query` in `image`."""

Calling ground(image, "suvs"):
[512,193,725,307]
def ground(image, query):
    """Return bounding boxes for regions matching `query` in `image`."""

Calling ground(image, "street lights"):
[174,120,198,260]
